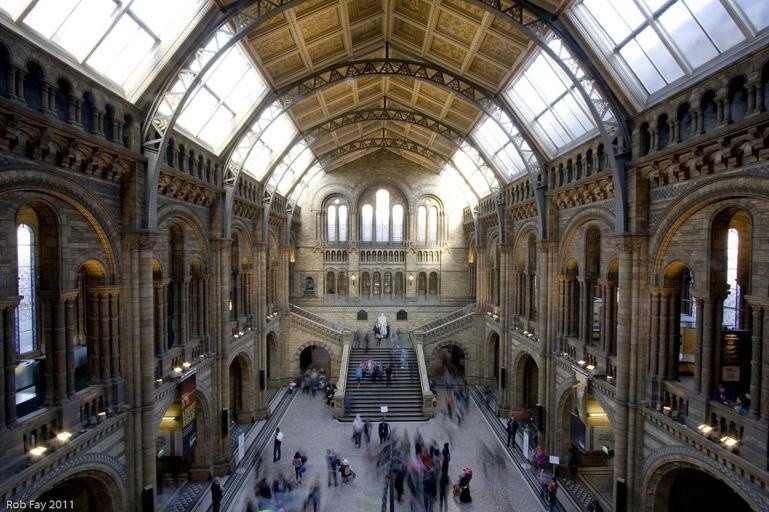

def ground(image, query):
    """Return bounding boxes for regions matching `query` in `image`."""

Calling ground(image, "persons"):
[585,500,601,511]
[241,414,472,511]
[342,322,416,389]
[211,475,224,512]
[732,395,749,416]
[715,383,733,406]
[377,311,387,335]
[288,365,336,407]
[534,444,579,511]
[506,415,520,448]
[426,348,492,425]
